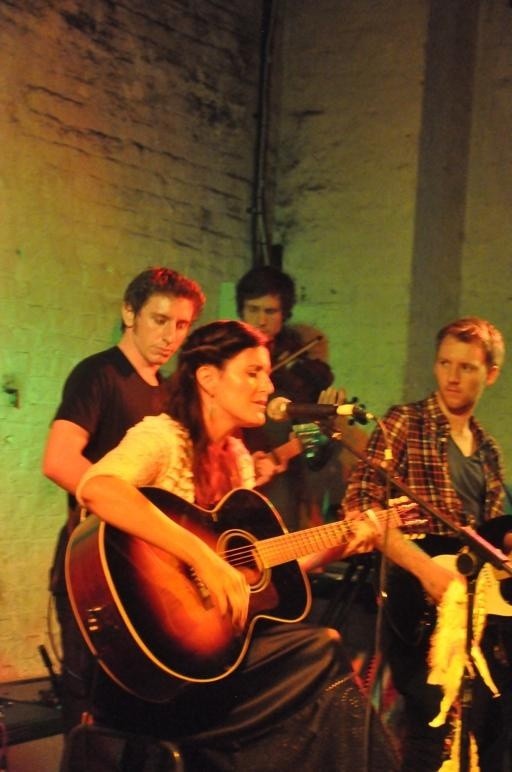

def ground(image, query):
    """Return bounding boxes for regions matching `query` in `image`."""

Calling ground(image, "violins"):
[268,329,376,430]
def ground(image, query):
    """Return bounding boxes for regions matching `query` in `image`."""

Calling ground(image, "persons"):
[75,320,401,771]
[235,265,349,533]
[42,265,205,772]
[339,315,511,771]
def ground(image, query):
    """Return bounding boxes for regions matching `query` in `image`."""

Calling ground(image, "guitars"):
[62,489,431,705]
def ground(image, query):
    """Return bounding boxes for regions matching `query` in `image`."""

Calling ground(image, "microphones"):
[266,397,374,422]
